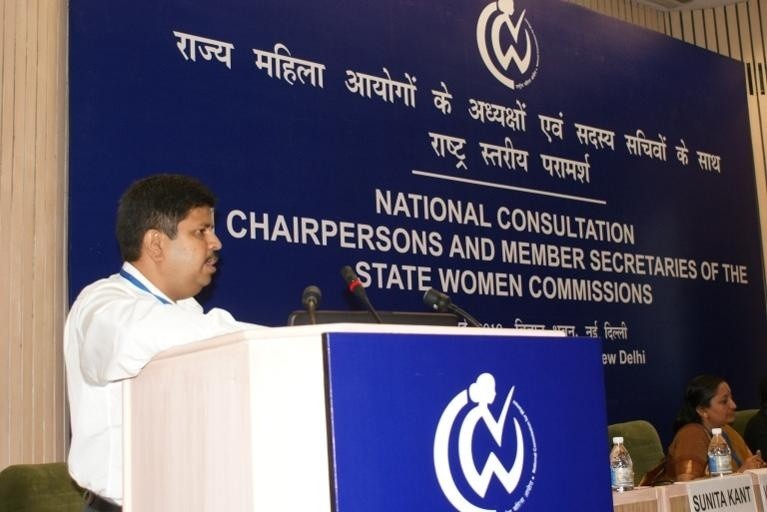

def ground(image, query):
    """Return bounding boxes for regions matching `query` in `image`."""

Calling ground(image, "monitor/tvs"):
[290,310,458,324]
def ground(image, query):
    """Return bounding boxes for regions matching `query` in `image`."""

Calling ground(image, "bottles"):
[705,427,733,478]
[607,436,634,492]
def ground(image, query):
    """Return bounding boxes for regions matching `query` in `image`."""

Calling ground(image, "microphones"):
[422,288,479,326]
[341,266,385,322]
[300,285,321,323]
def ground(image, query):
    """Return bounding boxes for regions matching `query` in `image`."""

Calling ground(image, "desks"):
[610,465,767,512]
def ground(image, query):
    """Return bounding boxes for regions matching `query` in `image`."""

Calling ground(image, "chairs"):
[606,415,663,485]
[0,452,102,512]
[729,405,759,447]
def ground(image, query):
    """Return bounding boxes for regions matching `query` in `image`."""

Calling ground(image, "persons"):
[743,384,766,467]
[666,373,763,482]
[63,171,263,512]
